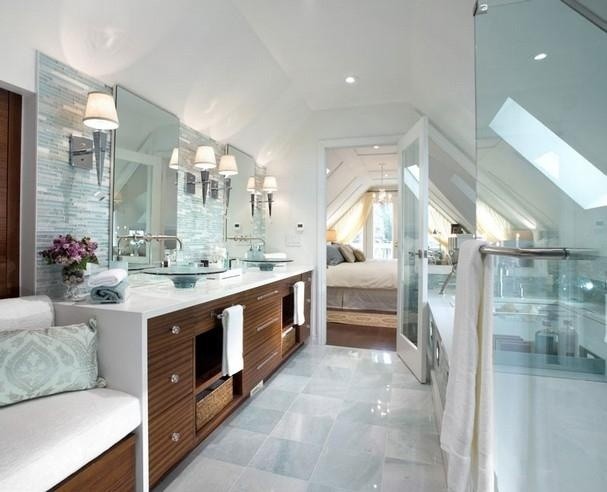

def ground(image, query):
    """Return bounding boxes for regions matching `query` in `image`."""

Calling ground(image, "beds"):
[326,257,398,315]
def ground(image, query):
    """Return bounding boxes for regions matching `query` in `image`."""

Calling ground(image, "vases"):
[63,272,82,301]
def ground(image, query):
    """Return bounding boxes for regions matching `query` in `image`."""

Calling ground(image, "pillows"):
[327,243,344,265]
[0,317,107,408]
[339,244,357,263]
[353,249,366,262]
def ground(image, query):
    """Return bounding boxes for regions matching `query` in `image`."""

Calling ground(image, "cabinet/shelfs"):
[146,270,314,491]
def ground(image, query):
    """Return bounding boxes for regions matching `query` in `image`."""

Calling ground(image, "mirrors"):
[223,143,256,242]
[109,82,180,270]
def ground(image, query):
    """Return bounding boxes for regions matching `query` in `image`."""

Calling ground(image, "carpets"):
[326,310,397,329]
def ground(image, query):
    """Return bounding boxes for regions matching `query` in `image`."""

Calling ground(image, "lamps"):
[256,176,279,216]
[184,146,216,206]
[209,155,238,208]
[326,230,336,242]
[246,177,256,216]
[70,91,119,186]
[169,148,178,183]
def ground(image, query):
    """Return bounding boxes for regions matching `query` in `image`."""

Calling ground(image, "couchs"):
[0,294,142,492]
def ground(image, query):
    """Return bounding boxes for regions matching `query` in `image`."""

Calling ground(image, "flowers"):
[38,233,99,273]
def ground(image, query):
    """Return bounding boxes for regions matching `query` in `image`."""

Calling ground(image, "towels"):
[439,238,495,491]
[88,268,128,305]
